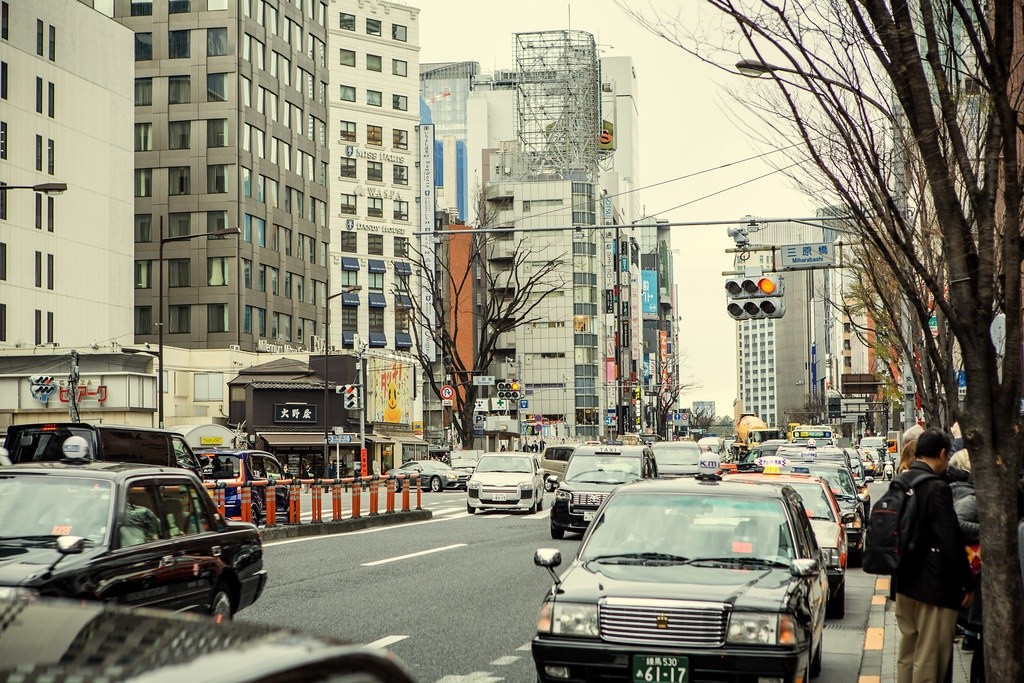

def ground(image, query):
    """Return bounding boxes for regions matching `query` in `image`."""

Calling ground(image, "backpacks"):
[863,472,938,574]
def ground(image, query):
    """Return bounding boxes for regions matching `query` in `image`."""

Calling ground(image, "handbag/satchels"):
[964,538,982,593]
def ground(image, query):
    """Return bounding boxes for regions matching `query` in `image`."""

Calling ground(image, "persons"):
[897,422,981,649]
[877,427,972,683]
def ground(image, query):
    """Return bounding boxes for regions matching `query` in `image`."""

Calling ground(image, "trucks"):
[449,449,485,475]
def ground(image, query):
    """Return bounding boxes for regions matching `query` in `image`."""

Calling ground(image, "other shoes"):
[961,635,976,654]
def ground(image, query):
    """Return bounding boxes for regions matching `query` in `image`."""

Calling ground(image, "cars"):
[0,585,416,683]
[384,460,473,493]
[648,412,896,620]
[1,461,268,622]
[531,475,829,683]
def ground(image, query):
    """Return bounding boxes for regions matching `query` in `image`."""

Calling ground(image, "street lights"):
[121,214,241,428]
[321,285,362,492]
[735,59,950,432]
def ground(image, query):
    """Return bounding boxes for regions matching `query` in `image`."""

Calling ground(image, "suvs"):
[194,448,295,528]
[465,451,545,514]
[541,443,582,493]
[547,443,659,542]
[6,421,206,486]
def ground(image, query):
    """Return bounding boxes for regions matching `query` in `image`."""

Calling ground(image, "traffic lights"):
[497,382,521,391]
[724,273,785,299]
[725,297,786,320]
[497,391,521,400]
[335,384,358,411]
